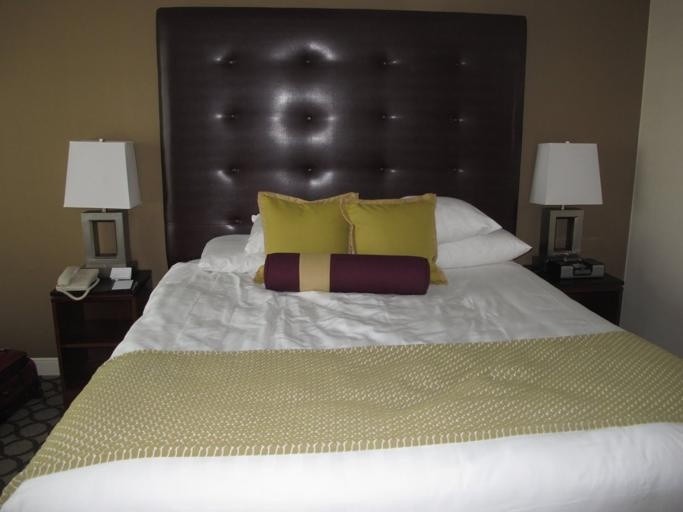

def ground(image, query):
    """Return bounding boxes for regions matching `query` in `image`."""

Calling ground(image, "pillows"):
[254,192,359,285]
[338,192,447,285]
[433,196,502,240]
[246,214,266,257]
[200,234,248,274]
[264,253,431,293]
[439,231,532,267]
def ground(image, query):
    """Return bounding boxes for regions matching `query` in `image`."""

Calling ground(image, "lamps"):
[63,141,141,211]
[529,141,604,211]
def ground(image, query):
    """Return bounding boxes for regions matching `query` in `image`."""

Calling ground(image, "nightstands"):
[50,268,150,404]
[526,260,624,327]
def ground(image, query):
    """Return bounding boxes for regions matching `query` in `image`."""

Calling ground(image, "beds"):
[1,256,682,512]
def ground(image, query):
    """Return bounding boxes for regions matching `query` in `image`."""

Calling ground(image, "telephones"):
[56,266,99,292]
